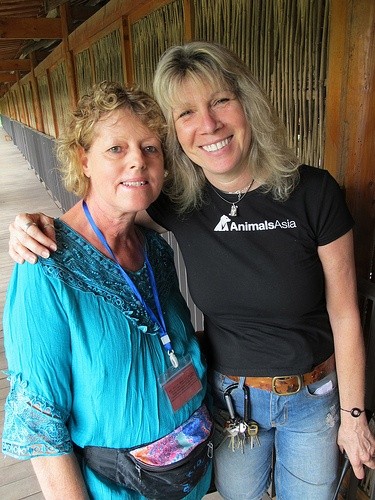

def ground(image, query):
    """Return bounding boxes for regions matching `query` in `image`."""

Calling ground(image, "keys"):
[226,418,261,456]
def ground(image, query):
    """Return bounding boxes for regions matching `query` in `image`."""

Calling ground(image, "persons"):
[1,84,217,500]
[7,38,375,500]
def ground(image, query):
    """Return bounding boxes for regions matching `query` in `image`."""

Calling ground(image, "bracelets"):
[338,407,368,417]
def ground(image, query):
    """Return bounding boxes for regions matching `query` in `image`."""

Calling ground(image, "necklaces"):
[205,177,255,218]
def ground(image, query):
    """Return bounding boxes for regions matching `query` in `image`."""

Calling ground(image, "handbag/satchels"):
[83,403,215,500]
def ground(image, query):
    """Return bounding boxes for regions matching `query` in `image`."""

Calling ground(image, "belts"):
[228,355,336,397]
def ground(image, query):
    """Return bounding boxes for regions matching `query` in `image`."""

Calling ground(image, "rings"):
[42,223,55,232]
[23,222,37,232]
